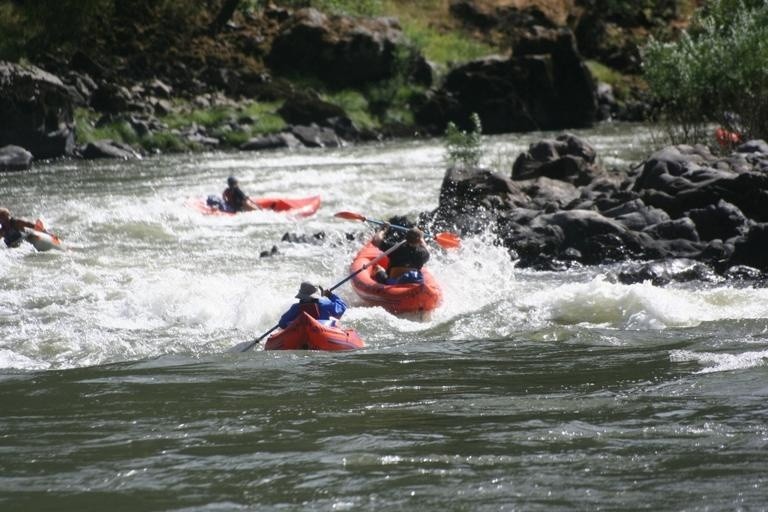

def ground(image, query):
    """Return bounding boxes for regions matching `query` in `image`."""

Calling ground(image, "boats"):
[716,127,752,149]
[349,229,444,312]
[20,218,67,252]
[264,310,363,351]
[189,195,322,217]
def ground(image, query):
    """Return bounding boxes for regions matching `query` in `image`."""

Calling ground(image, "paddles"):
[334,211,459,248]
[225,239,408,354]
[33,220,60,240]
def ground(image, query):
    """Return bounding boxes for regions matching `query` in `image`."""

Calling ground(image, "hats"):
[295,280,322,299]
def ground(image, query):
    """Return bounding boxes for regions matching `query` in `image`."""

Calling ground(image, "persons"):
[277,278,347,330]
[220,176,267,211]
[370,220,431,285]
[0,206,48,246]
